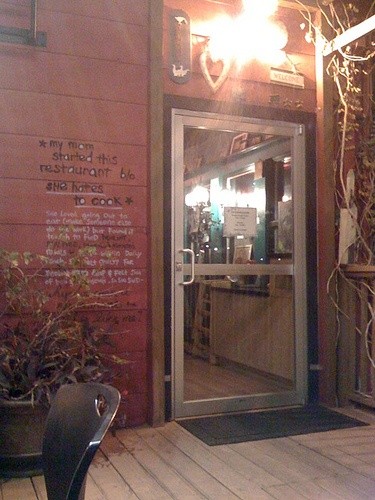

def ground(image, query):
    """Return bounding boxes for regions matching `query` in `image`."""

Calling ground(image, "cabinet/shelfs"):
[211,253,296,389]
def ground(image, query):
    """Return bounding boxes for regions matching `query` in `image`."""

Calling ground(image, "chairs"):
[39,382,123,500]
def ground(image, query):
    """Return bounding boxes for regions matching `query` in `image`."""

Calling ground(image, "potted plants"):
[0,224,136,477]
